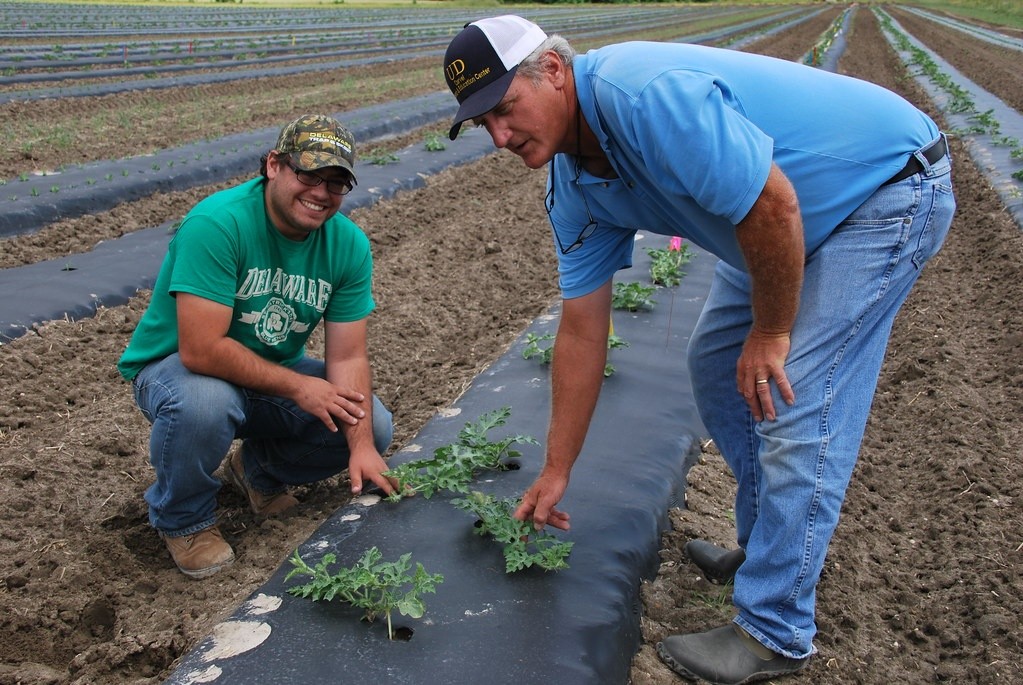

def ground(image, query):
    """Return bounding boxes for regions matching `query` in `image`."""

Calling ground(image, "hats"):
[443,14,548,141]
[276,114,358,187]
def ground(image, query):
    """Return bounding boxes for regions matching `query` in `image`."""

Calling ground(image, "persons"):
[117,114,417,579]
[444,14,955,685]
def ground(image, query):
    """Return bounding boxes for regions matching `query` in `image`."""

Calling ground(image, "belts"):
[880,134,946,188]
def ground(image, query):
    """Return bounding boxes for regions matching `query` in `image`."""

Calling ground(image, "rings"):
[756,380,768,384]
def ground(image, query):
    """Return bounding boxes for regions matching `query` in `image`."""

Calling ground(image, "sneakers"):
[229,441,299,518]
[164,524,235,580]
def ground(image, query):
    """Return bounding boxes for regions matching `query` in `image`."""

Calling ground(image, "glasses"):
[285,160,353,195]
[544,168,599,254]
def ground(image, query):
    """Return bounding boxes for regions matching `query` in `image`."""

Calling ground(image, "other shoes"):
[684,540,747,586]
[654,625,810,685]
